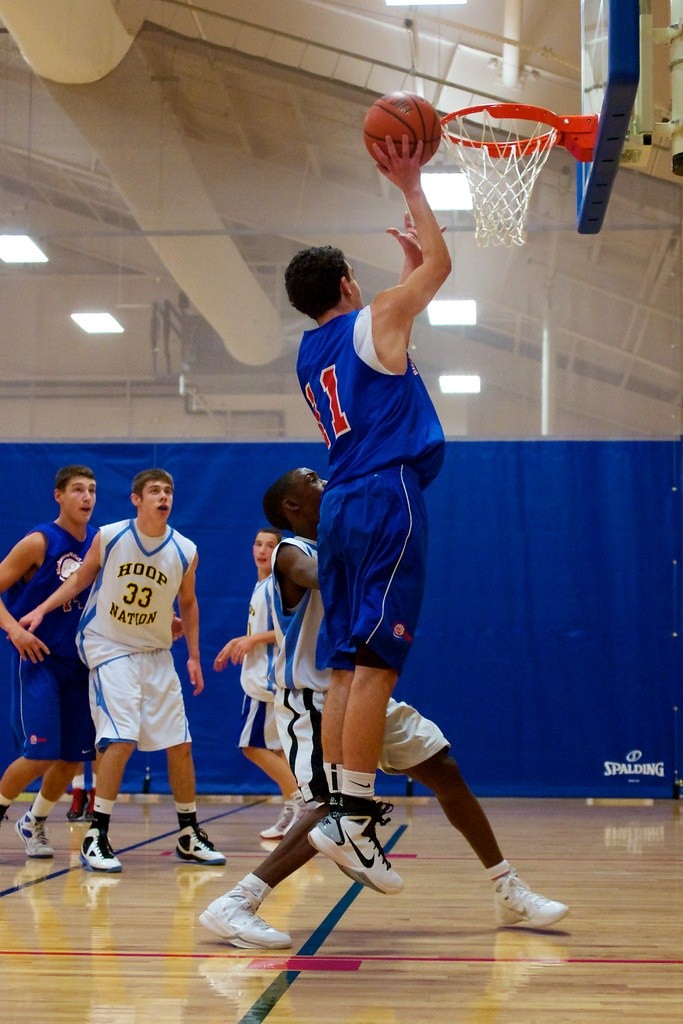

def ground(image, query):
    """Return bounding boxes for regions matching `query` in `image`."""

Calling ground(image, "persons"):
[7,469,228,873]
[213,528,324,842]
[0,465,186,859]
[285,134,452,897]
[67,744,104,821]
[199,467,572,951]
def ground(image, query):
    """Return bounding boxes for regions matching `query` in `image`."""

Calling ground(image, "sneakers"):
[77,826,124,875]
[199,888,291,950]
[15,809,54,860]
[260,794,316,841]
[494,870,568,930]
[65,785,106,822]
[306,799,403,897]
[177,823,226,868]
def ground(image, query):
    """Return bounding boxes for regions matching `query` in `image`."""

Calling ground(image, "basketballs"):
[363,90,441,166]
